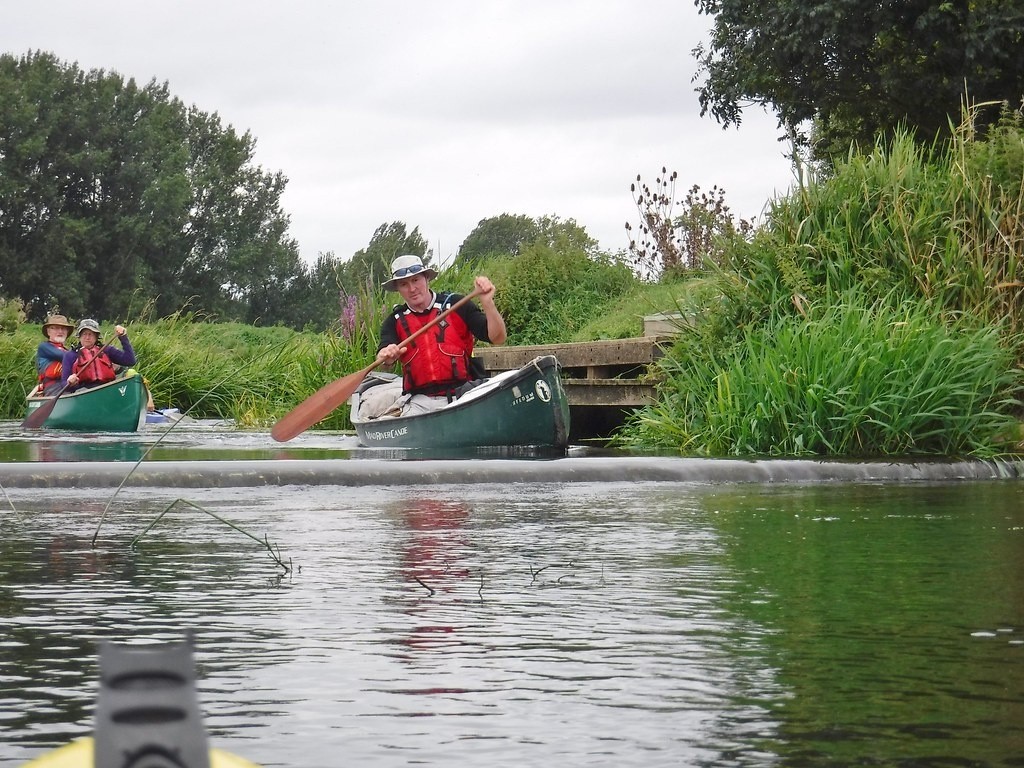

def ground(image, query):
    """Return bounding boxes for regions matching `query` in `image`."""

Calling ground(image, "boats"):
[26,373,151,433]
[145,407,180,424]
[350,355,570,461]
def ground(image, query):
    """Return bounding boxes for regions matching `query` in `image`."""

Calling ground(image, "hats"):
[75,318,101,338]
[41,315,74,339]
[381,254,439,292]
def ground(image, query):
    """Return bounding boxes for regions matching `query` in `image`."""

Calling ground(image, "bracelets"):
[382,358,398,365]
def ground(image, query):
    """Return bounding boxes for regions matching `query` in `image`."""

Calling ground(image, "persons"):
[36,315,119,396]
[376,254,507,418]
[61,318,136,393]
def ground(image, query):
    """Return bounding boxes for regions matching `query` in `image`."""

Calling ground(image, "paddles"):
[21,328,125,428]
[152,409,194,423]
[270,281,489,442]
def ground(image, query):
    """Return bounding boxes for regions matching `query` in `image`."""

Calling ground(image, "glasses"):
[391,265,427,280]
